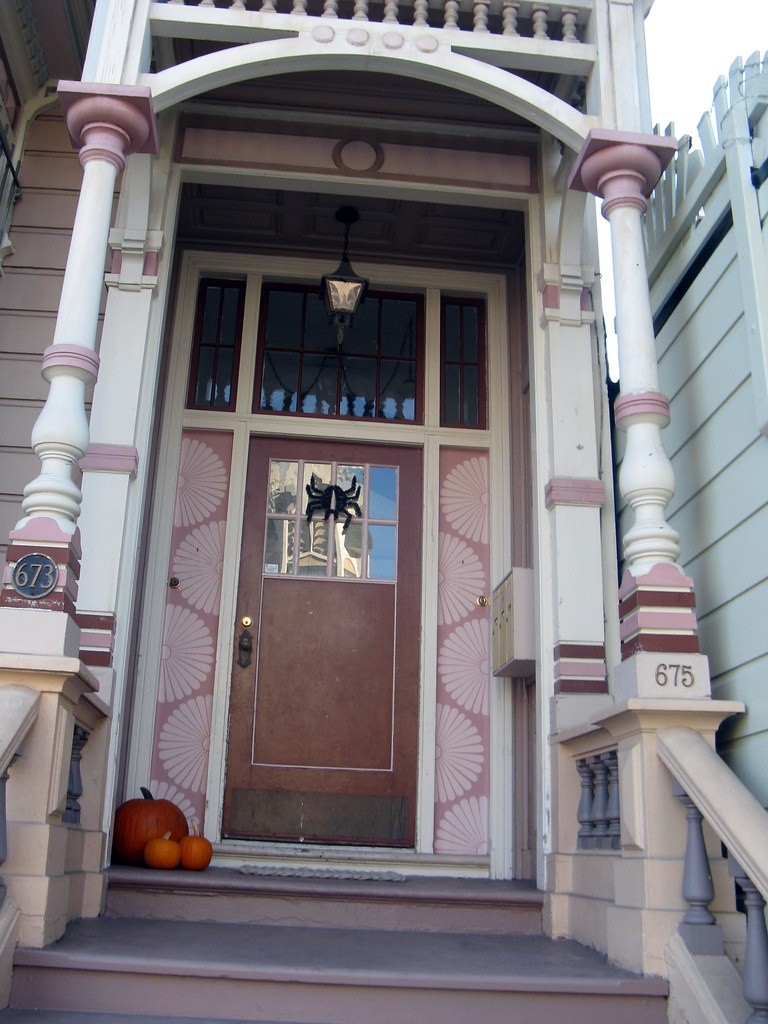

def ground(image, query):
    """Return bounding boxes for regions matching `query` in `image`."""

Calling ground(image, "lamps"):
[318,204,369,352]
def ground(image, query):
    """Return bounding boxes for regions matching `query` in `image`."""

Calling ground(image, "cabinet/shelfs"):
[491,567,535,679]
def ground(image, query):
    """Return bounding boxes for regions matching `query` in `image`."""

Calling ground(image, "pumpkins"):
[115,787,212,871]
